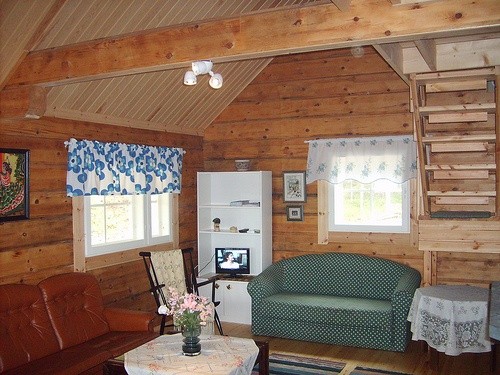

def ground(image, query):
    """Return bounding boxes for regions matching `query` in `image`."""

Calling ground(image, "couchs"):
[247,252,422,352]
[0,272,160,375]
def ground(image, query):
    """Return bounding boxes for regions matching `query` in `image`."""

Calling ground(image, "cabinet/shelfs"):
[190,170,272,325]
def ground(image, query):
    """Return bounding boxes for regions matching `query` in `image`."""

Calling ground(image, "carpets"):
[252,351,413,375]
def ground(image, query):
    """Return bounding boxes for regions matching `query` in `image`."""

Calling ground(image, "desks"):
[407,286,495,357]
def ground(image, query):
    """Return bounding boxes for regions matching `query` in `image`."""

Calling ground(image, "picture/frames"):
[282,170,306,204]
[286,204,303,222]
[0,147,30,222]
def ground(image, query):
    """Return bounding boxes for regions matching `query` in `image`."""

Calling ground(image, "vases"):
[180,320,201,356]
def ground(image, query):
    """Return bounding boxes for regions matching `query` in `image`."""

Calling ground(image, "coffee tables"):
[104,333,269,375]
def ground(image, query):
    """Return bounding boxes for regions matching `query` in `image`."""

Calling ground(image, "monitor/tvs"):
[214,248,250,278]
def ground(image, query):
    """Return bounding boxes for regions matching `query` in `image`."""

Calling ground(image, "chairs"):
[139,247,224,336]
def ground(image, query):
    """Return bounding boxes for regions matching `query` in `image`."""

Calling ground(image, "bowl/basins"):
[235,160,249,171]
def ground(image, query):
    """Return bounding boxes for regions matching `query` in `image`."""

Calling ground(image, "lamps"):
[183,60,223,90]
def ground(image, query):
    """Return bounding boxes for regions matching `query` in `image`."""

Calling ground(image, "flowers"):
[166,287,215,336]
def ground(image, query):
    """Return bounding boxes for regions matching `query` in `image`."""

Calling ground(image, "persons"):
[219,252,239,269]
